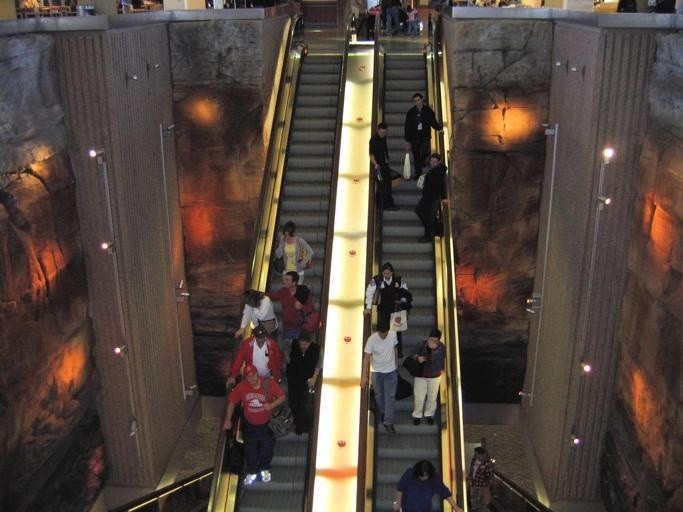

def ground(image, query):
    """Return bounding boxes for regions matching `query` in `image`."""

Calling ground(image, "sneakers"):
[261,469,272,483]
[244,473,256,485]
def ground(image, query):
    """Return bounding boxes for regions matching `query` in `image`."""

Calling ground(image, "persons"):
[407,328,446,425]
[467,447,492,510]
[284,332,323,434]
[263,271,313,345]
[362,261,407,359]
[223,364,286,485]
[224,326,282,389]
[370,122,400,212]
[392,460,463,511]
[275,221,313,286]
[19,0,44,18]
[380,1,419,37]
[404,94,443,180]
[414,153,449,242]
[358,320,399,433]
[232,289,278,344]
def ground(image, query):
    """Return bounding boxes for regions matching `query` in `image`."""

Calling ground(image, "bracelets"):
[312,373,319,377]
[393,501,401,504]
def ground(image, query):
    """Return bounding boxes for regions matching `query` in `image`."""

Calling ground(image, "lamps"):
[159,122,198,400]
[88,147,143,460]
[518,123,559,405]
[568,146,615,448]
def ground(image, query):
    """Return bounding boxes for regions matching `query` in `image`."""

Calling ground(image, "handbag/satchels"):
[274,257,285,273]
[297,258,313,270]
[402,355,424,377]
[301,310,320,333]
[267,401,294,438]
[389,303,408,332]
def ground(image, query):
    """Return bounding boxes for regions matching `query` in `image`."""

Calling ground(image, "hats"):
[244,364,258,377]
[253,325,268,337]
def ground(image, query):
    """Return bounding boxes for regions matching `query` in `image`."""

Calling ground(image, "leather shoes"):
[414,417,420,425]
[384,205,400,211]
[417,236,431,242]
[384,424,395,433]
[426,416,433,425]
